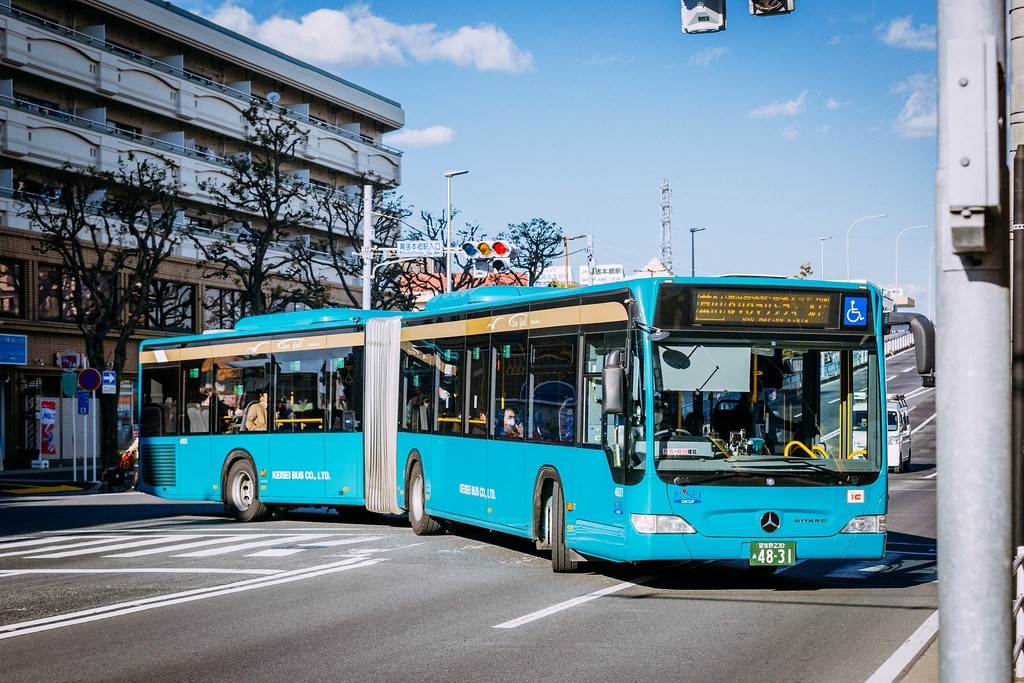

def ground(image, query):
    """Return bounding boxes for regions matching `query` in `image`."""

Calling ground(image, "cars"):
[493,375,576,436]
[528,353,573,374]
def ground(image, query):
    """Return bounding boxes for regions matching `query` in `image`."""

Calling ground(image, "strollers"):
[97,452,134,493]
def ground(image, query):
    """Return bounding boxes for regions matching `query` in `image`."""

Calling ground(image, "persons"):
[853,414,867,430]
[405,369,544,440]
[165,379,285,431]
[634,391,676,437]
[735,376,816,454]
[121,436,139,491]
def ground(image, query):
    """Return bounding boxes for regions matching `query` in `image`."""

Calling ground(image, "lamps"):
[35,357,45,365]
[6,377,11,383]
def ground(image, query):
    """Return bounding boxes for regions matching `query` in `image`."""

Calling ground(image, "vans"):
[850,396,913,471]
[757,410,782,442]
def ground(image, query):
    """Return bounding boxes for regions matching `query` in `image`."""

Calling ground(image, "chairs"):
[239,400,260,432]
[342,410,358,433]
[187,403,207,433]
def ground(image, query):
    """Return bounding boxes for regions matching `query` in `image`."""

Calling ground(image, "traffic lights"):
[462,240,511,258]
[747,1,797,20]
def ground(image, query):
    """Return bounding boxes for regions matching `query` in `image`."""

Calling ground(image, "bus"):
[224,360,322,425]
[135,274,935,573]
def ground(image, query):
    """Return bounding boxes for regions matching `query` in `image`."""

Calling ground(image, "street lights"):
[895,226,931,309]
[846,213,889,280]
[563,237,574,287]
[445,170,471,290]
[821,237,830,280]
[690,226,705,277]
